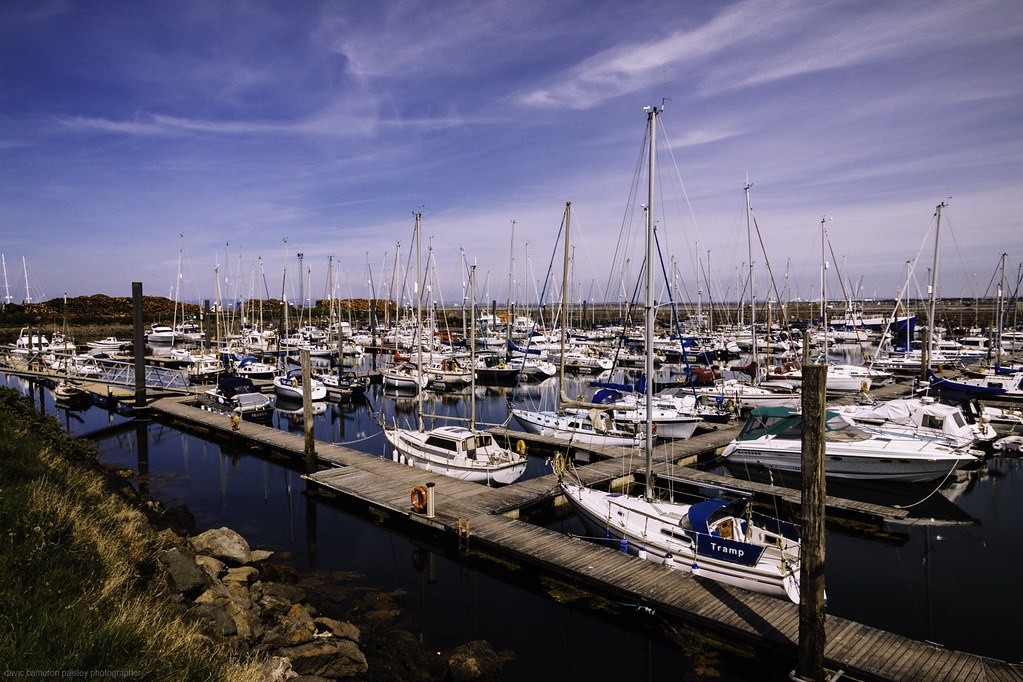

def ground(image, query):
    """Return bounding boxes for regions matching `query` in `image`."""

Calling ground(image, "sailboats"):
[551,99,830,608]
[728,458,985,648]
[4,171,1022,486]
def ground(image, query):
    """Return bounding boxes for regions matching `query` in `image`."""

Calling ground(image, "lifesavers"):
[230,415,235,427]
[292,380,298,386]
[499,363,504,368]
[410,486,427,509]
[761,368,767,377]
[555,453,564,471]
[517,439,525,454]
